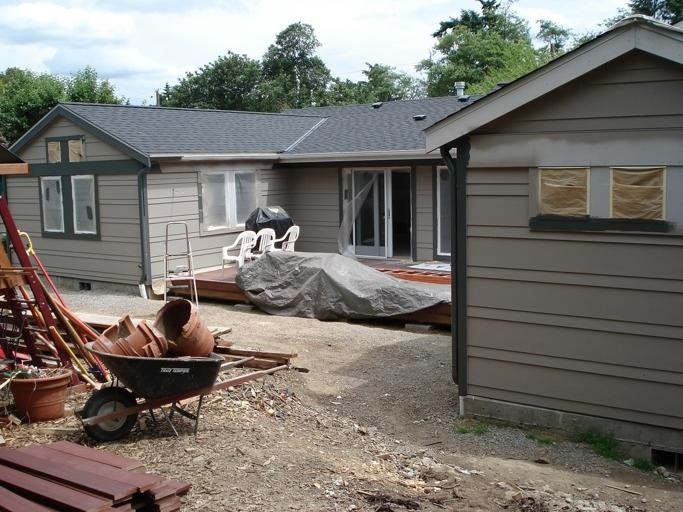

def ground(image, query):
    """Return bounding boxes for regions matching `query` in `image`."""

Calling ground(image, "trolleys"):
[73,340,289,443]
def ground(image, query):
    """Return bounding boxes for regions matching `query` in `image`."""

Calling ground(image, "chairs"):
[221,224,300,269]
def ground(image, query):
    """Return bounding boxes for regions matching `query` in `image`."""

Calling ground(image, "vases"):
[3,366,73,422]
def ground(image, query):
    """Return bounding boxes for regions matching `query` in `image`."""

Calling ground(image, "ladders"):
[0,196,80,387]
[164,221,199,315]
